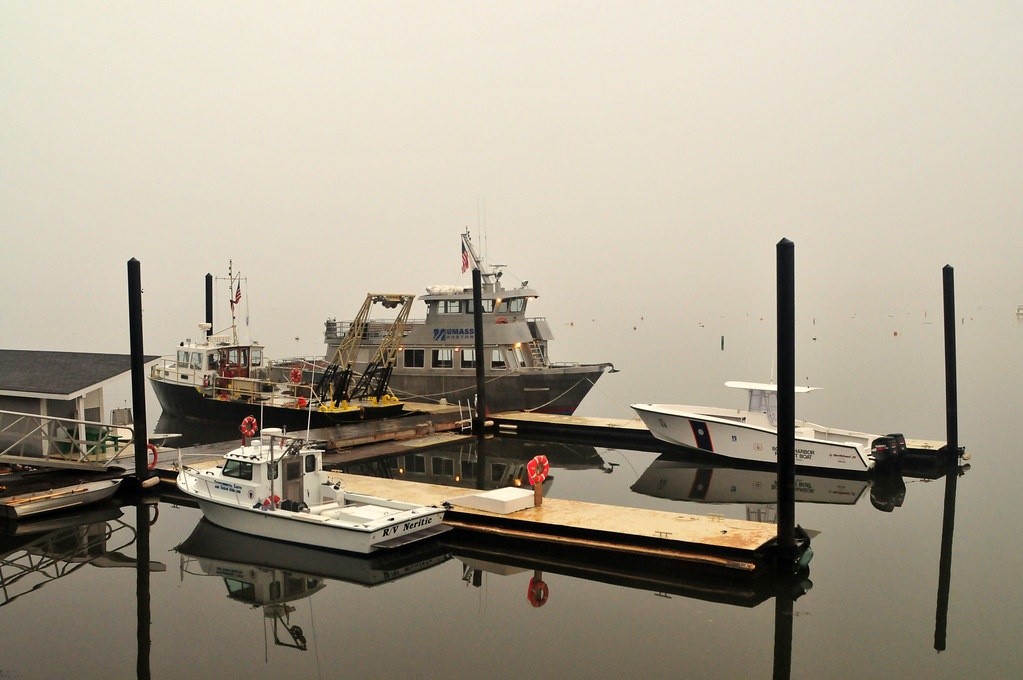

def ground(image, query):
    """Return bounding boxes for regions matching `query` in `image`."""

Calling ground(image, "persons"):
[209,354,213,363]
[219,354,226,369]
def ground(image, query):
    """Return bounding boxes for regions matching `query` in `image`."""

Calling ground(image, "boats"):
[168,516,453,653]
[0,476,124,520]
[146,256,410,429]
[176,397,455,555]
[626,375,906,475]
[312,226,620,417]
[0,503,130,546]
[629,446,912,525]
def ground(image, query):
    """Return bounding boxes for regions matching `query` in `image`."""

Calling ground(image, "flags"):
[462,240,469,273]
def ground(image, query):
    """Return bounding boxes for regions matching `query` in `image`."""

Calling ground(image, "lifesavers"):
[527,454,549,483]
[241,416,258,436]
[528,580,550,609]
[291,369,302,383]
[148,503,157,525]
[148,445,158,468]
[265,496,282,509]
